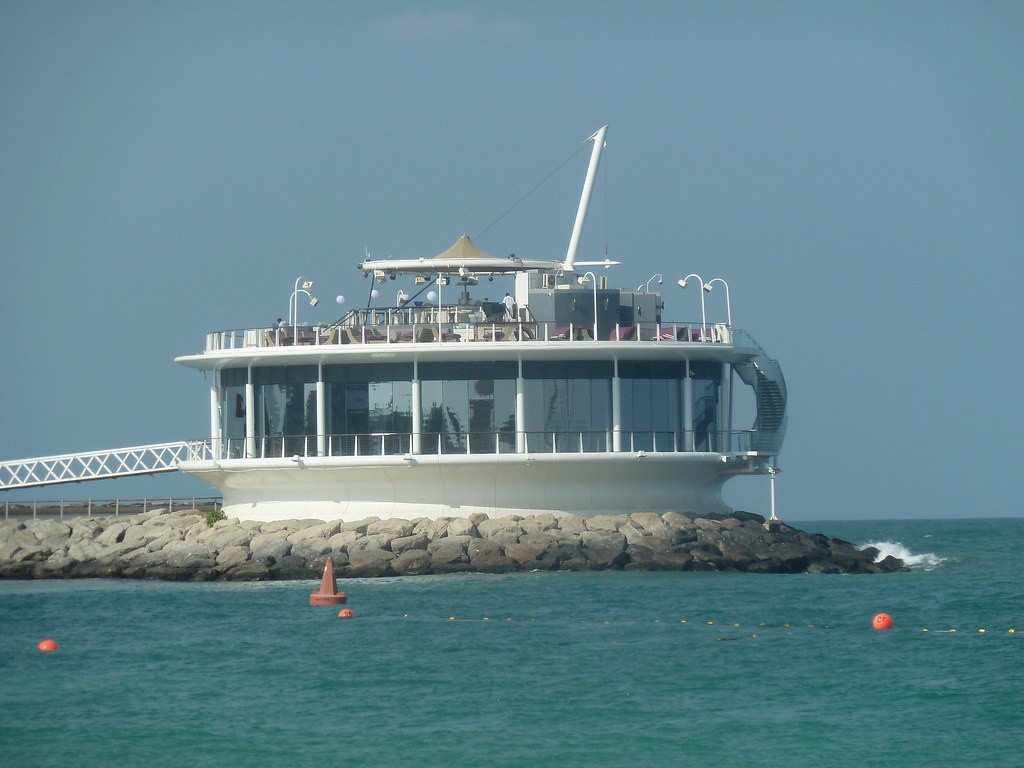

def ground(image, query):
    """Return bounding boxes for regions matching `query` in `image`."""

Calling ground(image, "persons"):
[503,293,516,322]
[277,318,290,331]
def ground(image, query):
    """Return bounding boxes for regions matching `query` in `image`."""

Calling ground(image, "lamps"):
[636,451,647,457]
[374,267,479,286]
[404,453,412,460]
[291,455,302,462]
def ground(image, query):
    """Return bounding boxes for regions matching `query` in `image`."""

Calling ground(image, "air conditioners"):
[543,274,555,289]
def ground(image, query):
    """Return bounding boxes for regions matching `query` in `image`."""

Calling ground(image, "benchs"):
[264,325,721,347]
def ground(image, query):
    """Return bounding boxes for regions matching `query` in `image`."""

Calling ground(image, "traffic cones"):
[309,553,346,605]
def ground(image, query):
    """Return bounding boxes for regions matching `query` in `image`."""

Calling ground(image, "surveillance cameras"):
[560,273,564,277]
[659,280,663,284]
